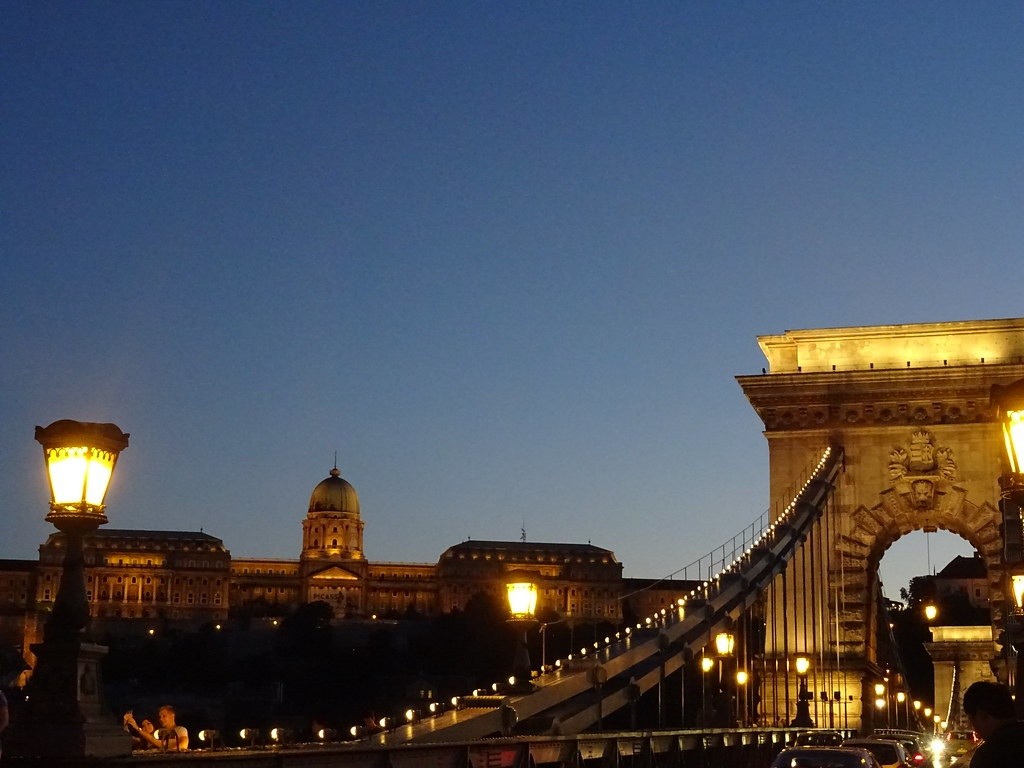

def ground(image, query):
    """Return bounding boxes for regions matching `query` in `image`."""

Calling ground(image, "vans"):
[945,730,975,754]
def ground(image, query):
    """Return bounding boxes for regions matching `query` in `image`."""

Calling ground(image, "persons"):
[128,705,189,749]
[0,690,10,732]
[122,714,158,750]
[962,682,1024,768]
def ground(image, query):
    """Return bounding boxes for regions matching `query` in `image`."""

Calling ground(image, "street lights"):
[505,577,540,695]
[715,632,738,727]
[934,714,940,735]
[0,420,134,768]
[791,658,816,727]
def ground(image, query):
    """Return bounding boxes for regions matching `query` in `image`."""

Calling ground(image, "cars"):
[793,732,844,748]
[867,734,933,768]
[769,745,882,768]
[838,739,918,768]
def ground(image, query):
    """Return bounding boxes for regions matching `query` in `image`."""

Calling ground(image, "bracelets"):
[138,727,142,732]
